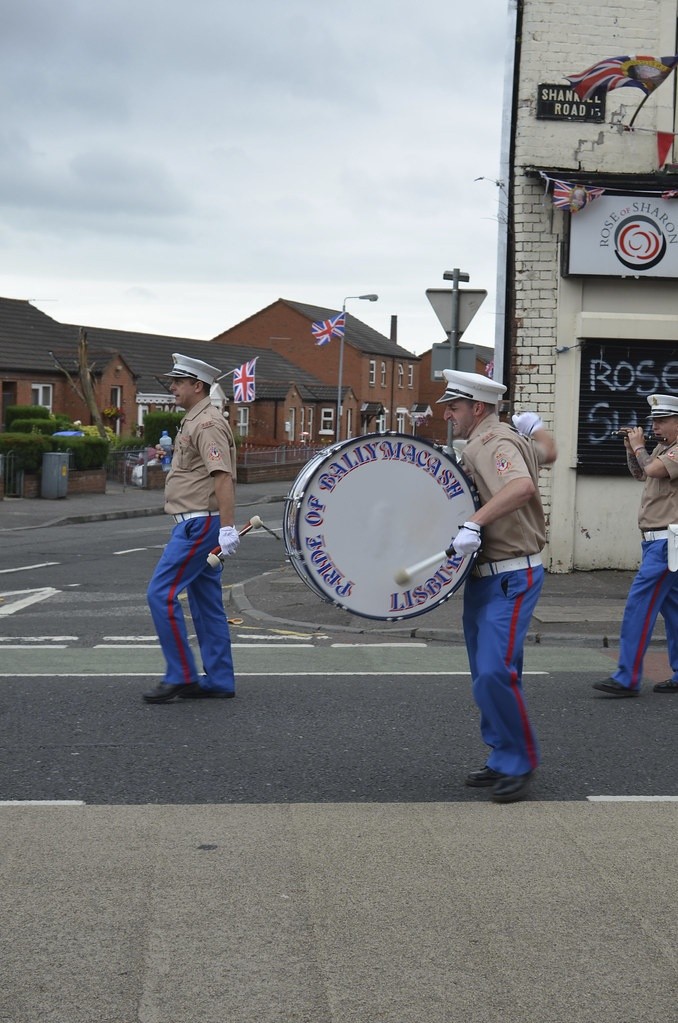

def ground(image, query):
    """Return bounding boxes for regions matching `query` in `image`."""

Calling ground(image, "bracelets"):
[636,447,645,451]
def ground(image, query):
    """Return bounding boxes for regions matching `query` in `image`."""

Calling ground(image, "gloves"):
[219,524,240,556]
[509,412,544,436]
[452,520,484,557]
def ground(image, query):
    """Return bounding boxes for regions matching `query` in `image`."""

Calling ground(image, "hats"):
[164,351,221,387]
[646,394,678,421]
[434,367,510,408]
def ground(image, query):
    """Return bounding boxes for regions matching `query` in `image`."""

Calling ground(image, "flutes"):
[611,430,668,442]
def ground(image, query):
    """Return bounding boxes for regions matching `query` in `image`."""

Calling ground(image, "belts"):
[642,530,671,543]
[470,551,543,579]
[172,511,221,524]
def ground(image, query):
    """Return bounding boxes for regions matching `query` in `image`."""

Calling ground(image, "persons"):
[437,368,558,802]
[593,395,678,695]
[143,353,240,701]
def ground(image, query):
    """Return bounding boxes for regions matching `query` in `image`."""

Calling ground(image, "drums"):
[280,428,484,622]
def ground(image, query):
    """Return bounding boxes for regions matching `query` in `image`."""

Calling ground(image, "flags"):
[233,358,256,403]
[552,181,604,212]
[312,314,345,346]
[561,56,678,101]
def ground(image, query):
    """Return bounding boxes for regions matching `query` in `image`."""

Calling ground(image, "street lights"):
[336,294,379,444]
[443,268,470,462]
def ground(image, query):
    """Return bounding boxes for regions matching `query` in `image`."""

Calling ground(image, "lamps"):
[223,411,229,418]
[113,365,123,379]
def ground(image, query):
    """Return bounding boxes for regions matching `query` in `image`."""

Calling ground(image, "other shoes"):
[177,681,235,699]
[488,772,535,803]
[463,765,510,788]
[593,676,640,697]
[142,678,198,703]
[654,678,678,693]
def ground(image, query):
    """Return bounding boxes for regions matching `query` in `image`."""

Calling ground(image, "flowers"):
[102,404,125,422]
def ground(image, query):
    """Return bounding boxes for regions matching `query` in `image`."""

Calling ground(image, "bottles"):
[159,431,172,471]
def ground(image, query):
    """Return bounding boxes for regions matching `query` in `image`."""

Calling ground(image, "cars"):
[439,439,471,478]
